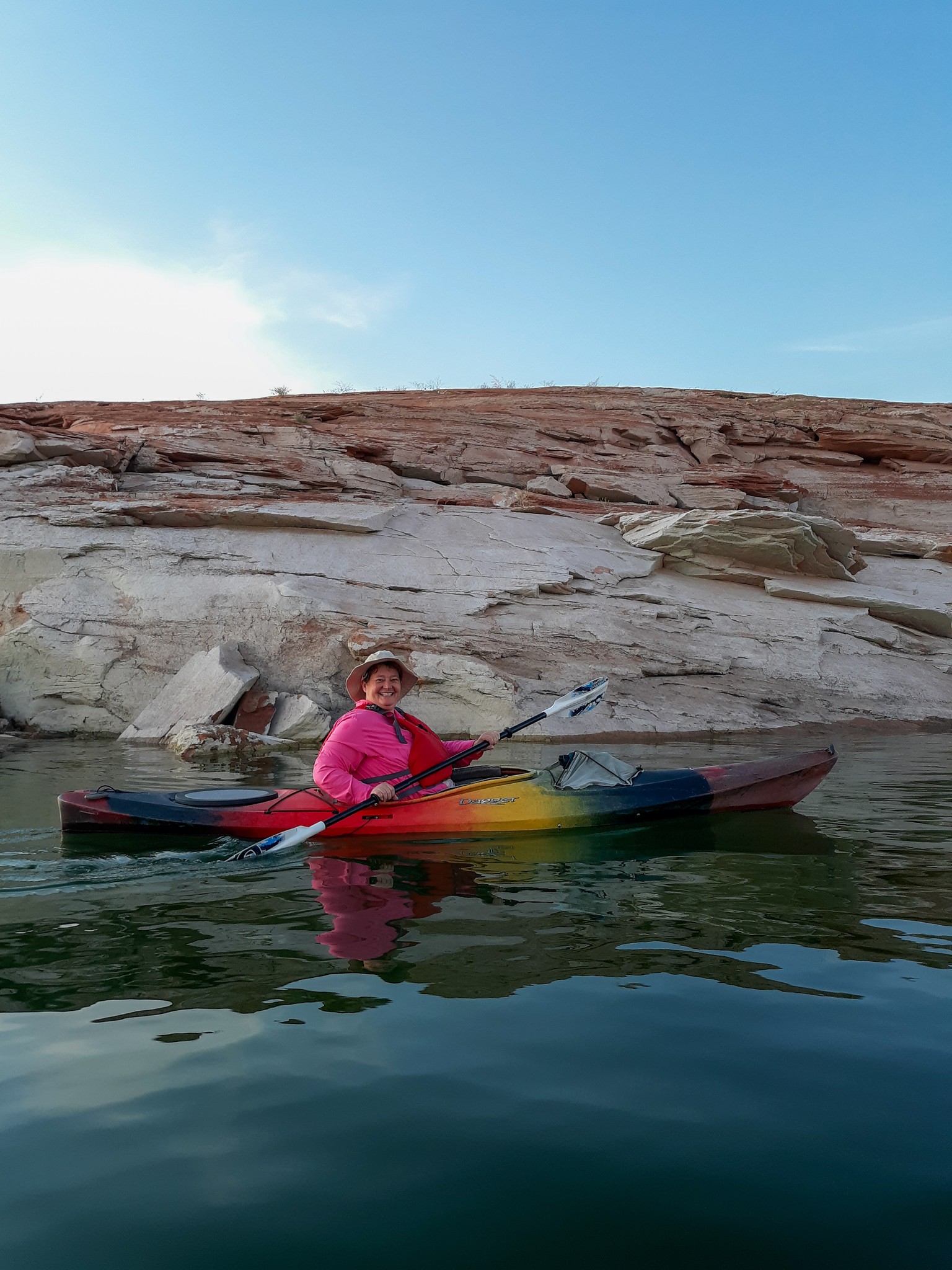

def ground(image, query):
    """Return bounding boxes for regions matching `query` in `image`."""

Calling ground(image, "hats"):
[346,650,419,703]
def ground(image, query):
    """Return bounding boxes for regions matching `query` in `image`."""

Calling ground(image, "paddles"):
[221,674,611,861]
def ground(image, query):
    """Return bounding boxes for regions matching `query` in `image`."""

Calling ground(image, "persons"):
[313,651,500,805]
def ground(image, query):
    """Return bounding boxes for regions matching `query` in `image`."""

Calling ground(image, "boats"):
[53,748,838,837]
